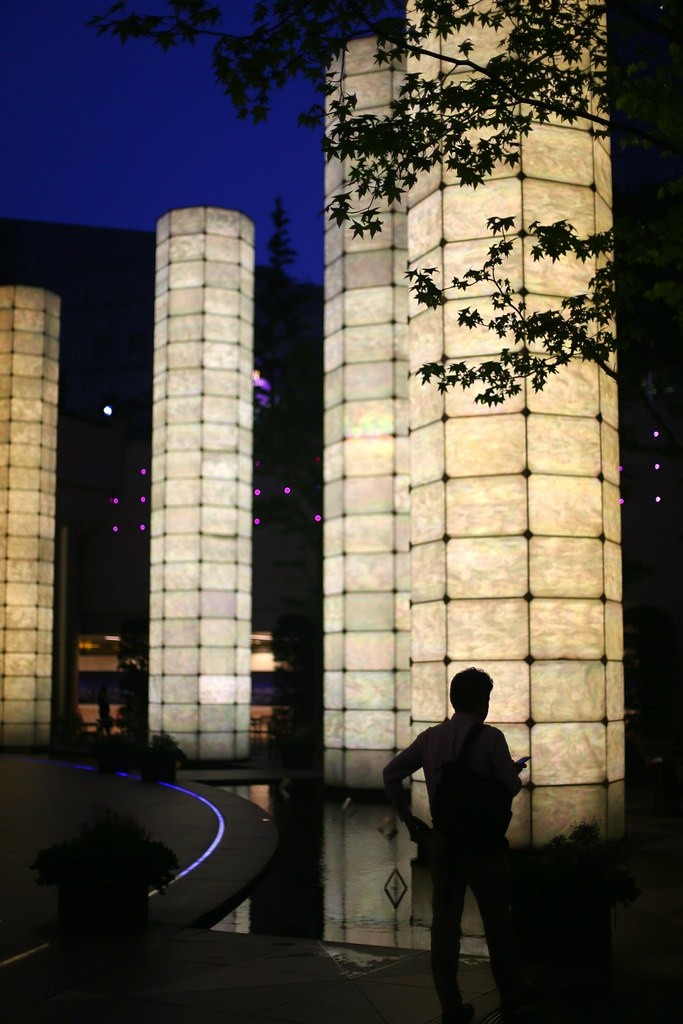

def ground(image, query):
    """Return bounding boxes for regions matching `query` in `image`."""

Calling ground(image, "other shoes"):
[440,1002,476,1024]
[501,980,545,1012]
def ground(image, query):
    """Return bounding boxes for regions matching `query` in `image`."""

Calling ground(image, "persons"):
[98,685,113,735]
[383,670,527,1024]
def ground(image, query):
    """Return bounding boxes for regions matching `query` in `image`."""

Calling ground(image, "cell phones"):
[516,757,531,765]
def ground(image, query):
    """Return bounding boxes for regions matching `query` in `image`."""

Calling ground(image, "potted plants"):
[93,730,138,775]
[26,802,180,938]
[507,816,640,969]
[140,732,186,785]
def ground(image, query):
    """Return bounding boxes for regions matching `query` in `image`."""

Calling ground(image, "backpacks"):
[429,722,513,908]
